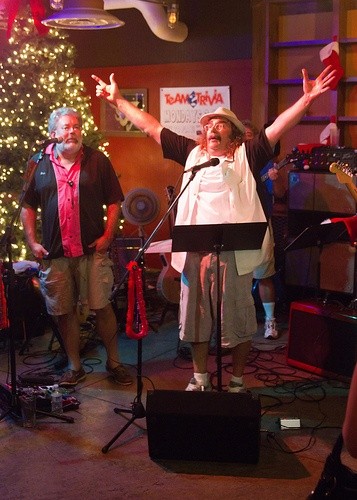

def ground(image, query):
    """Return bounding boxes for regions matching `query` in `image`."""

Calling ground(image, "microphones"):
[32,136,61,155]
[184,157,221,174]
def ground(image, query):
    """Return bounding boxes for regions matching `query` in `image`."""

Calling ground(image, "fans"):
[123,188,160,274]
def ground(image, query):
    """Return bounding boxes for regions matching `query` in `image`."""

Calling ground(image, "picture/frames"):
[99,88,148,138]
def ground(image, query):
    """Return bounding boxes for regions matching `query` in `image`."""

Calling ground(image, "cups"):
[21,396,36,428]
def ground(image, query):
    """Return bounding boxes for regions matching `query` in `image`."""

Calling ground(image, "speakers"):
[286,170,356,215]
[142,389,260,477]
[106,237,143,305]
[280,235,356,295]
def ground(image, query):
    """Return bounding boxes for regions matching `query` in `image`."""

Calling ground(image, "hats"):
[200,107,246,135]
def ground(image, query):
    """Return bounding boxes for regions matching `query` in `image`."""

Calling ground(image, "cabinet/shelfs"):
[252,0,357,159]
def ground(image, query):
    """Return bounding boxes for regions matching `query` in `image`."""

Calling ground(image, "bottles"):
[51,384,63,415]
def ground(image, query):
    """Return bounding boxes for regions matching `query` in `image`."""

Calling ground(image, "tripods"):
[99,167,196,454]
[0,146,73,425]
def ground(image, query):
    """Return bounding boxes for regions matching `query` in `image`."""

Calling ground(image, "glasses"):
[203,123,231,132]
[56,125,83,132]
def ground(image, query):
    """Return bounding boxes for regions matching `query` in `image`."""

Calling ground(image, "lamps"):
[41,0,125,30]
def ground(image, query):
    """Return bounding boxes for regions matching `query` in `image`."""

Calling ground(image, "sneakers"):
[264,317,279,338]
[228,381,245,392]
[59,367,87,385]
[105,360,133,385]
[185,378,212,392]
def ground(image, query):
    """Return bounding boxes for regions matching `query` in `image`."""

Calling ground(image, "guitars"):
[156,185,182,304]
[260,150,303,184]
[330,160,357,204]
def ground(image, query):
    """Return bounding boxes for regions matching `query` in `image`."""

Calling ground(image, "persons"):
[344,363,357,459]
[91,65,336,394]
[19,107,133,386]
[234,120,280,339]
